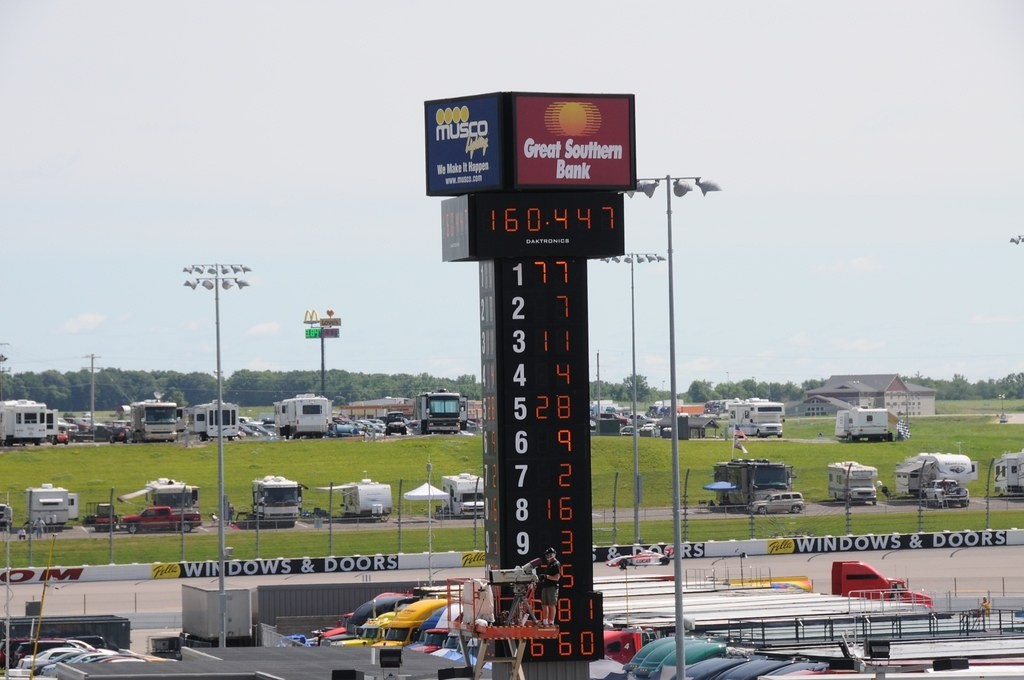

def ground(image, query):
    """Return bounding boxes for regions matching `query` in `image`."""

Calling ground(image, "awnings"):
[313,483,358,496]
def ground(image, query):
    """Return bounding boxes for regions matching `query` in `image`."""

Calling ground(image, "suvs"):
[745,491,807,514]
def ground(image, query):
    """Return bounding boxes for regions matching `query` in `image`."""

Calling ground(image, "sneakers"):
[538,624,555,628]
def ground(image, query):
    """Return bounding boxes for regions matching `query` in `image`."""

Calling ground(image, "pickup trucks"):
[116,506,203,535]
[921,479,969,508]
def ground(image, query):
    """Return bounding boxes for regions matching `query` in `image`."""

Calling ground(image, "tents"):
[404,483,451,520]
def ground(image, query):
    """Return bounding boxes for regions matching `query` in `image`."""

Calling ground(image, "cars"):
[328,410,420,436]
[233,414,285,440]
[600,413,632,427]
[48,415,134,446]
[0,578,180,680]
[628,414,655,427]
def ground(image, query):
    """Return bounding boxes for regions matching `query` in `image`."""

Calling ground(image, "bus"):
[130,402,181,443]
[273,393,333,440]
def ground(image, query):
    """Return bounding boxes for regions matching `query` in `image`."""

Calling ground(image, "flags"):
[735,424,749,442]
[735,438,748,454]
[895,419,911,439]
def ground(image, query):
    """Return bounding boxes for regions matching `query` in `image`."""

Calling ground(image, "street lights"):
[617,174,723,679]
[724,371,732,400]
[998,394,1008,423]
[751,375,756,398]
[180,260,254,650]
[662,379,666,414]
[596,251,666,540]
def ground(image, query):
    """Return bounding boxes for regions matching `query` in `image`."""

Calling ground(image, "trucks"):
[314,479,392,519]
[115,476,199,512]
[711,459,797,511]
[827,460,879,505]
[992,448,1024,499]
[728,397,786,438]
[835,405,889,441]
[0,399,59,445]
[302,557,1024,680]
[412,387,463,435]
[440,473,486,520]
[252,476,308,528]
[894,452,979,500]
[186,399,240,441]
[23,483,79,532]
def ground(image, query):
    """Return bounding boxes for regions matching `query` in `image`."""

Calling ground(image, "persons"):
[521,546,560,627]
[980,597,990,616]
[32,517,46,538]
[17,527,26,539]
[23,520,34,533]
[226,502,234,526]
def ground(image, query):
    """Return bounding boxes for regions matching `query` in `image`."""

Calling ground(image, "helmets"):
[543,547,557,554]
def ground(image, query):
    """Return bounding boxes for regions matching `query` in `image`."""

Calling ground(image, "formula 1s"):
[606,550,672,569]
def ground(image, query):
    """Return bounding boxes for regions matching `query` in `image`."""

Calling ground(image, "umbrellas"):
[702,480,741,505]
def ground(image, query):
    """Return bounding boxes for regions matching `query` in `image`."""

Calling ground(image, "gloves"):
[538,574,547,582]
